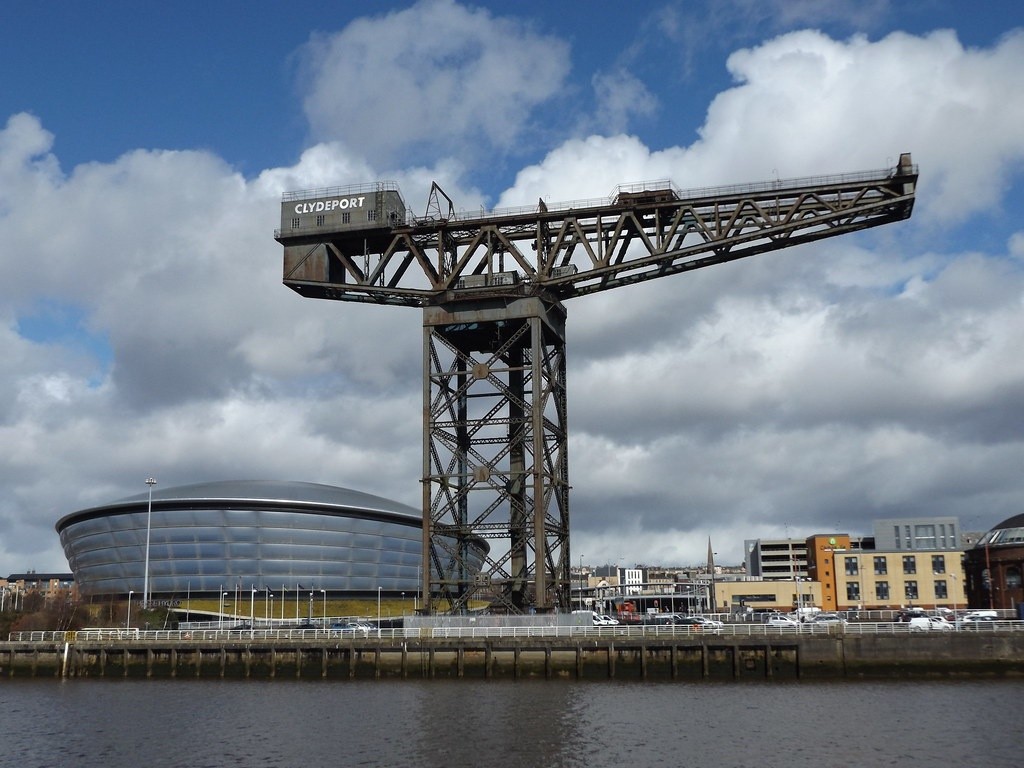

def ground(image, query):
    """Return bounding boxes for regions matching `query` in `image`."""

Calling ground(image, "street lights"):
[296,583,305,625]
[127,590,134,637]
[142,476,158,609]
[265,584,272,626]
[251,583,260,630]
[377,586,383,640]
[579,554,584,612]
[281,584,289,625]
[219,585,231,635]
[320,589,327,634]
[234,584,240,628]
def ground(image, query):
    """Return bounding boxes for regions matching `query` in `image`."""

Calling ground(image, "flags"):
[253,585,258,592]
[267,585,271,592]
[298,584,304,589]
[284,585,288,592]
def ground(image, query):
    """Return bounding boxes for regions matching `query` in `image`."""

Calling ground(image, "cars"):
[600,615,619,625]
[617,613,725,633]
[765,606,848,628]
[892,607,1000,626]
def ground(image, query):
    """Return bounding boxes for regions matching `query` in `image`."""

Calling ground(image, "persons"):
[801,616,805,623]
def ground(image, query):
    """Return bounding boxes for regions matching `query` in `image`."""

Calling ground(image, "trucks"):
[907,618,954,633]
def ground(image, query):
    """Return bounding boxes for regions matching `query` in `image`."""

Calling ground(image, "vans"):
[572,610,610,630]
